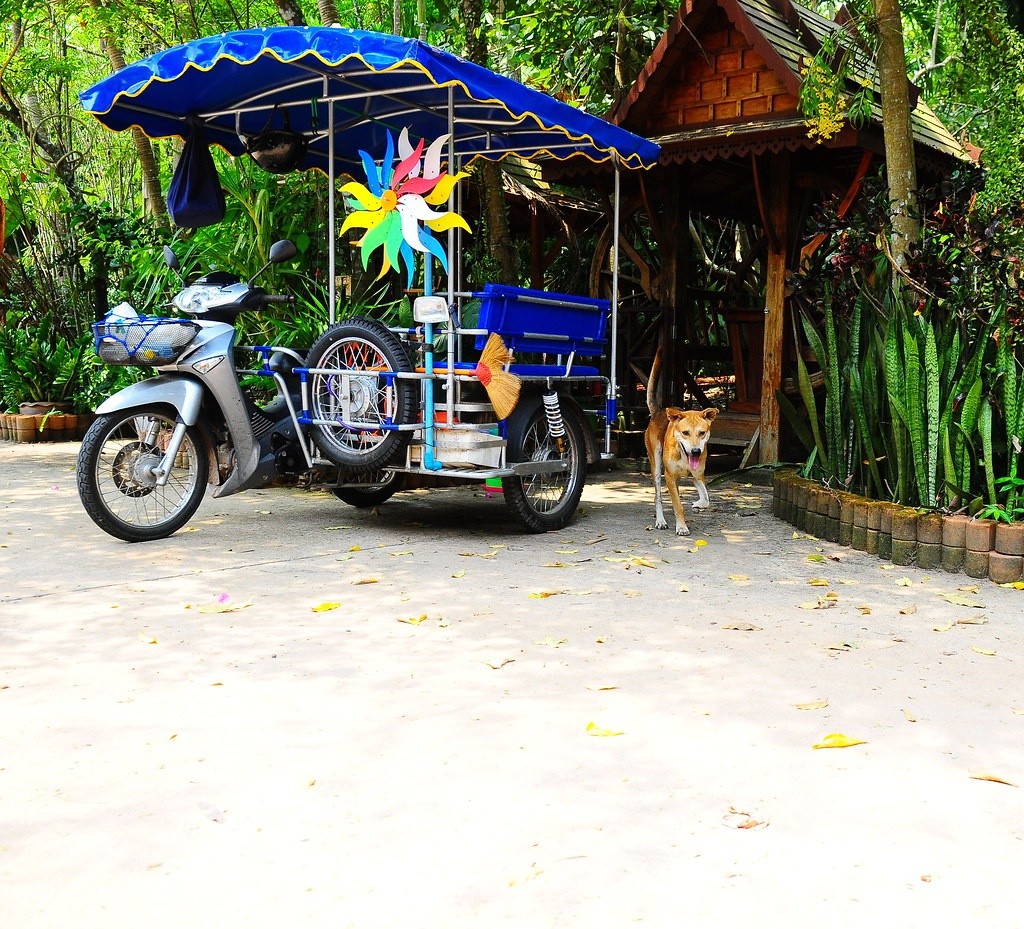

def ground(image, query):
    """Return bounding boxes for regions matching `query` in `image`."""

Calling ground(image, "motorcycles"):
[76,240,613,544]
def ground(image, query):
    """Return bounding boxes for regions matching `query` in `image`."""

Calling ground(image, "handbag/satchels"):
[166,142,225,227]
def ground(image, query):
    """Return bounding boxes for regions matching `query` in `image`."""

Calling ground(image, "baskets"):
[90,315,203,365]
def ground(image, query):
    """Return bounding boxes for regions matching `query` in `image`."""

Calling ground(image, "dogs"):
[644,404,719,535]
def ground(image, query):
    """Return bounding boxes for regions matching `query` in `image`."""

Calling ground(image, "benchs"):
[414,283,611,377]
